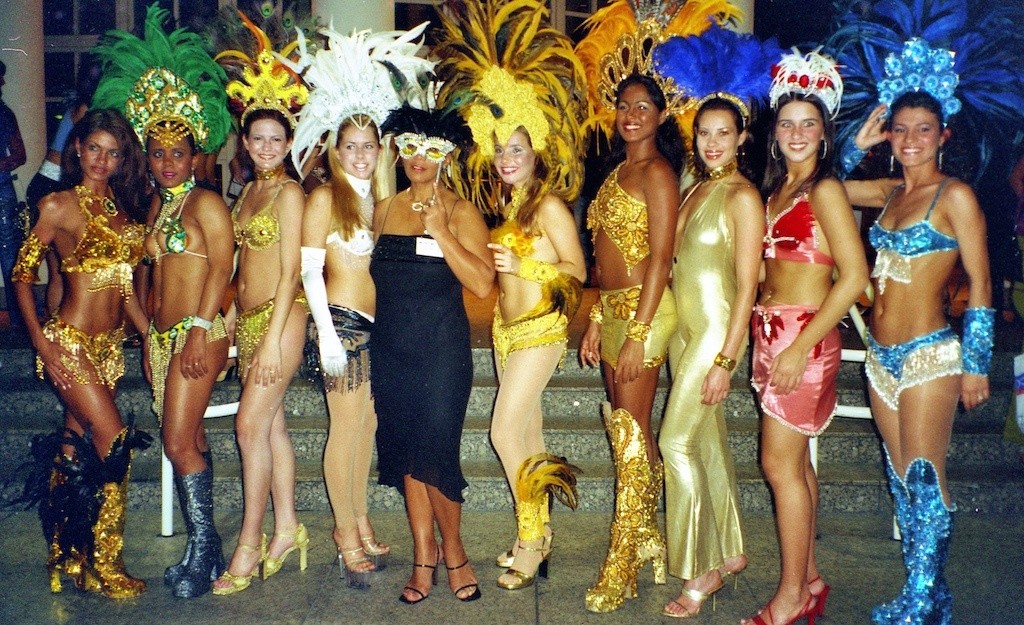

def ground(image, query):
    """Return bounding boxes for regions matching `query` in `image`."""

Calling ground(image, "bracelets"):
[624,320,653,342]
[714,353,738,371]
[587,303,603,323]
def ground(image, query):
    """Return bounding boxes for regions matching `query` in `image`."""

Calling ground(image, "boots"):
[46,437,104,594]
[164,479,197,588]
[897,459,956,625]
[870,442,918,623]
[82,423,145,599]
[584,398,668,612]
[171,468,223,598]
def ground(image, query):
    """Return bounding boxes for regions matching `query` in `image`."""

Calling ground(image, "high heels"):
[444,559,482,601]
[361,532,390,571]
[399,545,440,604]
[719,564,747,591]
[740,589,820,625]
[498,490,553,591]
[213,532,272,597]
[660,579,725,618]
[497,536,521,567]
[805,576,831,616]
[252,523,310,578]
[336,541,375,590]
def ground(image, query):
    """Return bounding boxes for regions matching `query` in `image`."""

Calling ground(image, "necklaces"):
[405,187,444,211]
[504,181,528,224]
[344,172,375,226]
[78,186,119,215]
[704,159,738,180]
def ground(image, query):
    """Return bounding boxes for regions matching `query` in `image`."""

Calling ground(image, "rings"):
[426,200,438,206]
[977,395,986,401]
[422,204,429,208]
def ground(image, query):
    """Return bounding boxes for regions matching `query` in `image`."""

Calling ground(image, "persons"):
[11,107,150,596]
[487,116,588,594]
[134,118,233,601]
[0,59,47,335]
[655,91,766,614]
[300,112,393,576]
[832,87,999,625]
[743,84,871,622]
[576,73,685,609]
[128,144,328,381]
[27,53,102,317]
[370,99,504,606]
[203,101,311,595]
